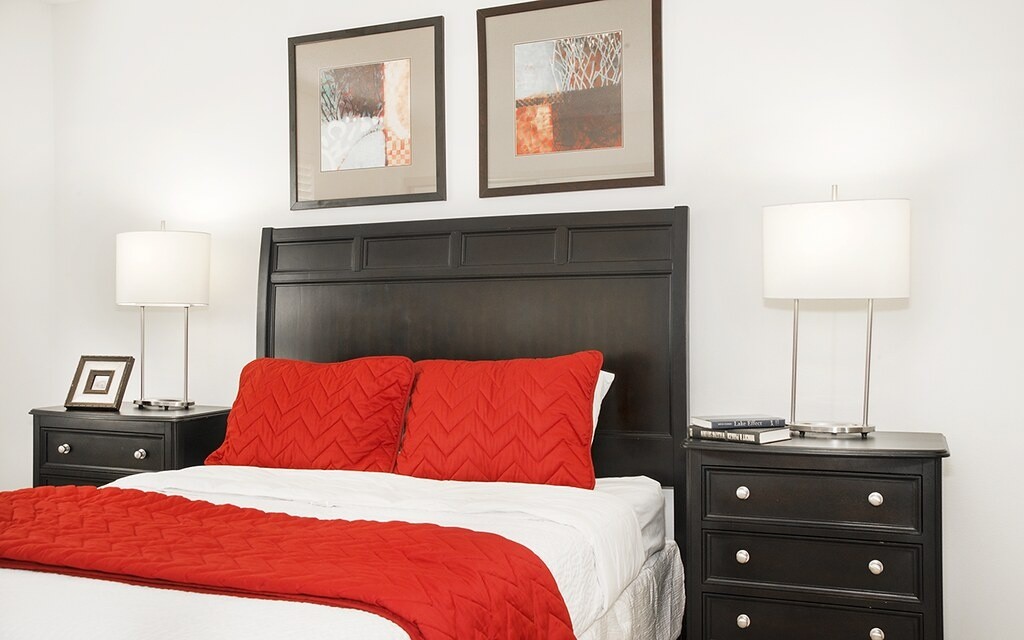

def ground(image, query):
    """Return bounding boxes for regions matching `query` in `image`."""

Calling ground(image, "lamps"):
[760,185,913,439]
[113,219,211,412]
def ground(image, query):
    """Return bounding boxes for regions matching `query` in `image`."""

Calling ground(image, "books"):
[688,415,791,445]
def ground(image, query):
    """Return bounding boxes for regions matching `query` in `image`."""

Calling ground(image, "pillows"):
[204,355,416,478]
[394,349,604,490]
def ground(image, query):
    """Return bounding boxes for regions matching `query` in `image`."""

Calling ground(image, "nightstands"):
[681,430,951,639]
[28,399,233,488]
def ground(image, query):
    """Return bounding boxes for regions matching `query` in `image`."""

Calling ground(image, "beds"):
[0,205,692,640]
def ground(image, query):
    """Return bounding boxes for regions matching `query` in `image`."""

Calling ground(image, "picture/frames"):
[476,0,665,198]
[287,15,448,212]
[62,355,136,410]
